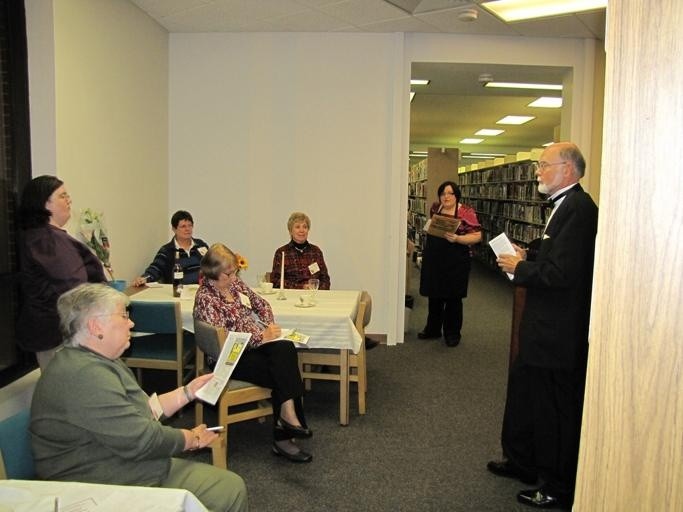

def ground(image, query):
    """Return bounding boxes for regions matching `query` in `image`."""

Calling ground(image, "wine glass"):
[306,278,321,302]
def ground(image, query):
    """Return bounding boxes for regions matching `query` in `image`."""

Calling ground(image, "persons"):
[487,141,599,508]
[417,181,484,347]
[28,282,248,512]
[132,209,209,286]
[193,243,313,463]
[270,211,380,350]
[14,175,107,376]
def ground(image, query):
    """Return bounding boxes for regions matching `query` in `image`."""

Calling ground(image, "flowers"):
[233,252,248,277]
[78,207,117,282]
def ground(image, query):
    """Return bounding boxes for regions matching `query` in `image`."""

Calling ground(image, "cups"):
[261,282,271,292]
[299,293,309,306]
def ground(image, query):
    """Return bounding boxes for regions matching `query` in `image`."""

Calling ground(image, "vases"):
[109,281,125,292]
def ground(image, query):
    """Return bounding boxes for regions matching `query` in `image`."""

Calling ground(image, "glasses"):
[441,192,456,195]
[535,161,567,168]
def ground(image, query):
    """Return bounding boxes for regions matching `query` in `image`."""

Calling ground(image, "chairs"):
[190,315,294,461]
[115,301,195,418]
[1,368,227,511]
[297,288,371,416]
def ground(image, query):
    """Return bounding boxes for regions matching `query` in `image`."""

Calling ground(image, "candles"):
[279,250,285,292]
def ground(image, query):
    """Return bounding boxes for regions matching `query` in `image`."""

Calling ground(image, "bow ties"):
[542,198,555,210]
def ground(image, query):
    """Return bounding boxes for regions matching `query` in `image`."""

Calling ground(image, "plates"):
[258,290,276,294]
[294,304,315,309]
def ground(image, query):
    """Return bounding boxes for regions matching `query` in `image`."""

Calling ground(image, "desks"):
[114,280,360,427]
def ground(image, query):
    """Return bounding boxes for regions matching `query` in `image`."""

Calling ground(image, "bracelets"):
[191,429,199,451]
[183,386,192,402]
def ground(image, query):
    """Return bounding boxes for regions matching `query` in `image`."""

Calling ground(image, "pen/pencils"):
[522,248,530,251]
[257,320,269,327]
[139,276,150,282]
[205,426,224,431]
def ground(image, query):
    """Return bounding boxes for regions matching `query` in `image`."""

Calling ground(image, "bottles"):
[170,251,185,297]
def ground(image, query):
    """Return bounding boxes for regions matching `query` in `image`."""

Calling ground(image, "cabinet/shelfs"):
[407,144,553,280]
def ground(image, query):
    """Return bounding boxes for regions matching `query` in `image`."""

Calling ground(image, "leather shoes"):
[270,440,313,464]
[442,332,461,347]
[486,459,539,488]
[517,486,574,511]
[273,413,313,439]
[417,327,442,340]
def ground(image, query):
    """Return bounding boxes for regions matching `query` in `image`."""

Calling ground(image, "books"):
[195,331,253,406]
[262,328,310,344]
[407,159,554,271]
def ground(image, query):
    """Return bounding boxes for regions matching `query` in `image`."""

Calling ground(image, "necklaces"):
[294,246,307,252]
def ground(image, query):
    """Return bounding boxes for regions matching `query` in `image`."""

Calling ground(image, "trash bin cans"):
[404,294,414,333]
